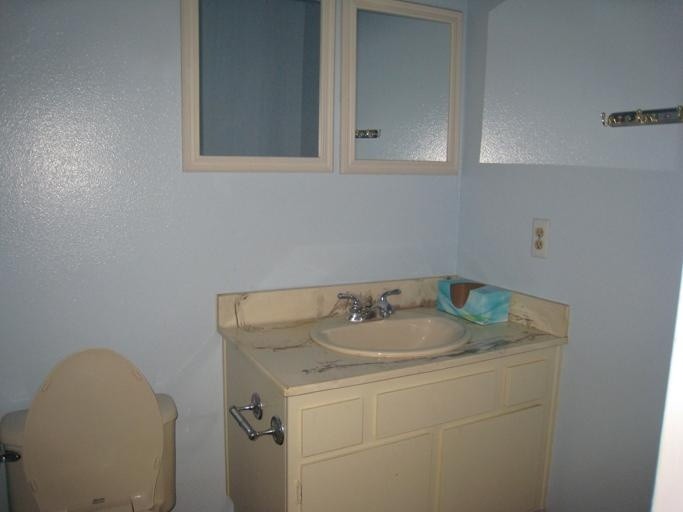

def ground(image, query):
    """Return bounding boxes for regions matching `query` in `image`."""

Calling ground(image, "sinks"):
[309,311,472,358]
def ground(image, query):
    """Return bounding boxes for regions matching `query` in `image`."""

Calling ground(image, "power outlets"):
[532,218,550,259]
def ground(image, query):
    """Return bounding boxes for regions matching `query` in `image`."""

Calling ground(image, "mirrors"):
[180,0,335,172]
[340,0,463,175]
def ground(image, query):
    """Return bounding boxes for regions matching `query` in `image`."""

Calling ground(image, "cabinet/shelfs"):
[224,340,564,512]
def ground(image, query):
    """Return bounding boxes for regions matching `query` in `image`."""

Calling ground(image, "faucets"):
[337,288,401,322]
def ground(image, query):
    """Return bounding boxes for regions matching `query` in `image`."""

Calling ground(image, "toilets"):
[0,346,178,512]
